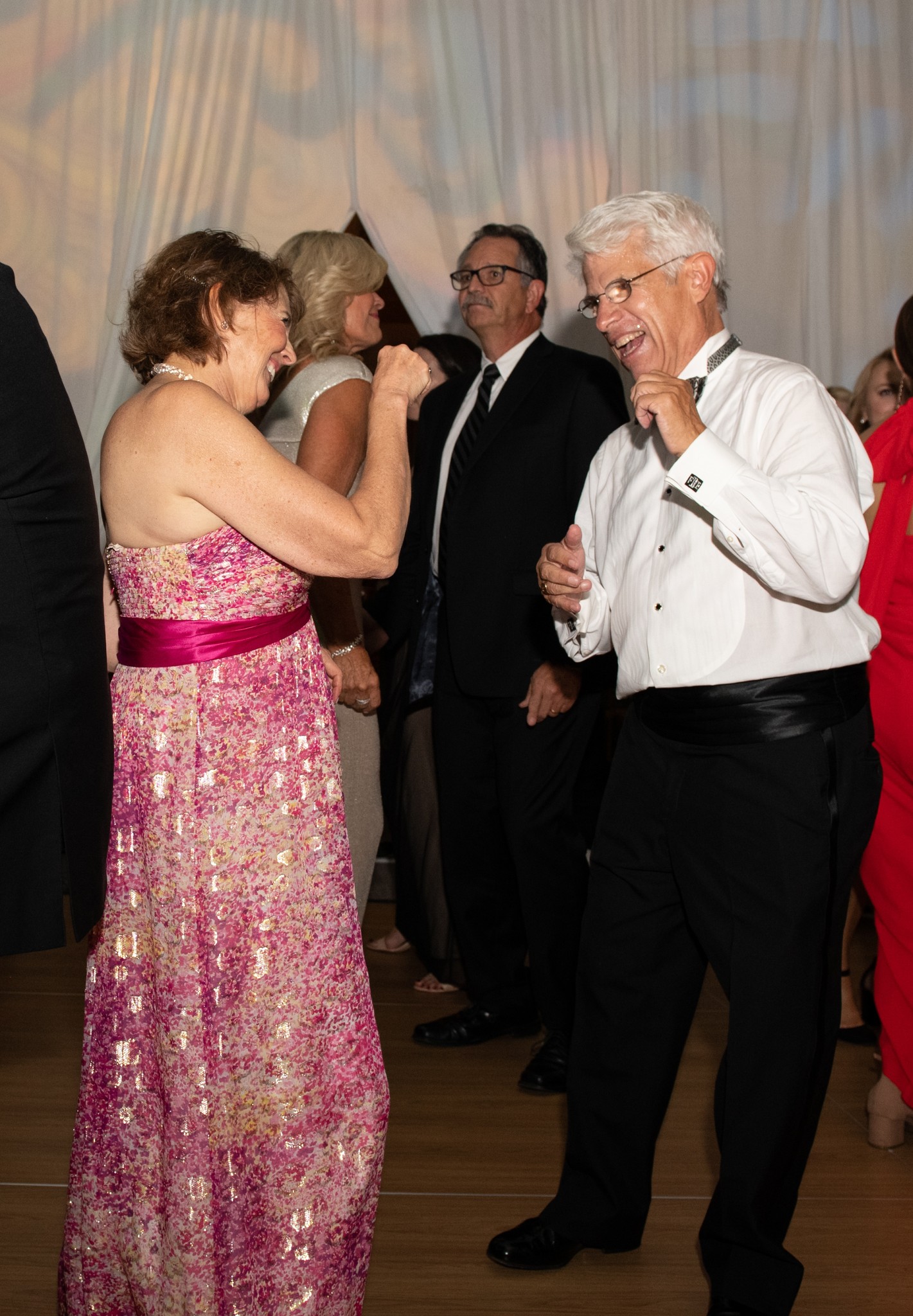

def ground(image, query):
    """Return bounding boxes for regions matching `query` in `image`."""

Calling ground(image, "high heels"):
[865,1072,913,1148]
[414,972,458,993]
[366,934,413,953]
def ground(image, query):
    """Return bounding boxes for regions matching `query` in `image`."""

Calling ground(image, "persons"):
[838,342,913,1056]
[481,182,890,1314]
[235,225,390,950]
[43,231,455,1314]
[822,381,870,433]
[862,293,912,1146]
[0,260,123,961]
[375,332,488,998]
[408,220,638,1100]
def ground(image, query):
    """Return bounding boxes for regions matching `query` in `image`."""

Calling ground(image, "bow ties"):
[685,333,745,405]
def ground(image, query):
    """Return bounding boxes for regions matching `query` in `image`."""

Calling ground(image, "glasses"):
[450,264,536,292]
[577,255,689,320]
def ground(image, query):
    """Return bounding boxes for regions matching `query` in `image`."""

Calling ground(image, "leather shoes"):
[486,1197,648,1272]
[412,1004,543,1047]
[517,1037,572,1097]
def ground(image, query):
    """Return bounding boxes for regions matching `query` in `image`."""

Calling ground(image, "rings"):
[355,697,373,706]
[550,709,560,715]
[428,366,432,374]
[540,580,549,595]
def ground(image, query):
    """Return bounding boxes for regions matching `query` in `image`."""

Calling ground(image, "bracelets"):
[331,635,364,658]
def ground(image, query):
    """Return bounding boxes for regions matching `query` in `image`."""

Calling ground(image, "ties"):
[437,362,503,602]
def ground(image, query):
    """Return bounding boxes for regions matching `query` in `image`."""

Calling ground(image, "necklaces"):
[150,362,196,382]
